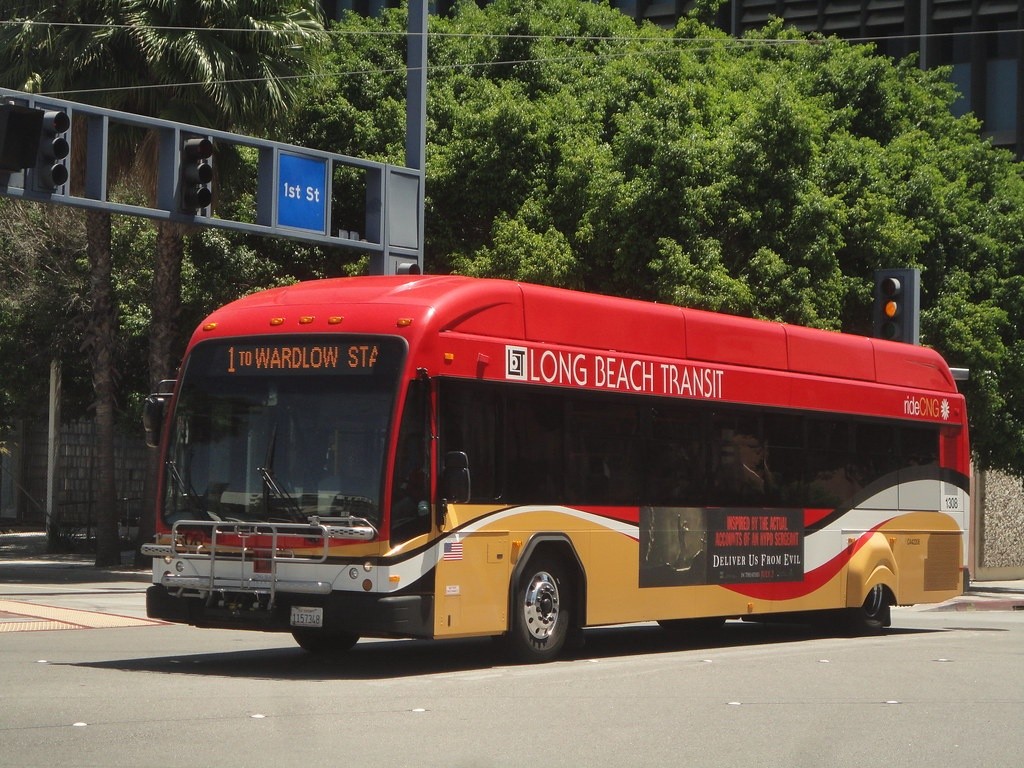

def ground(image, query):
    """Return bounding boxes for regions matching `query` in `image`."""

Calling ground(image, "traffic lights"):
[872,268,921,347]
[179,126,215,218]
[29,99,72,195]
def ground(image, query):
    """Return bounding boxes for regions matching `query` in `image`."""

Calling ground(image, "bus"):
[144,275,973,666]
